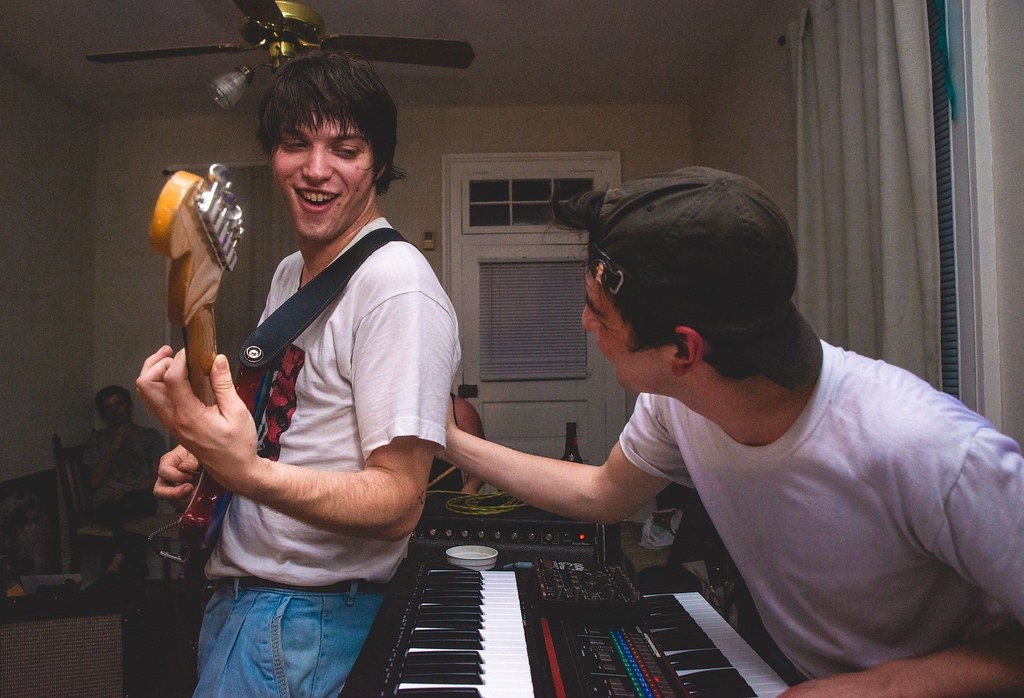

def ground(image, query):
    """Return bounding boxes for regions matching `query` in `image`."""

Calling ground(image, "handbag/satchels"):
[638,509,684,550]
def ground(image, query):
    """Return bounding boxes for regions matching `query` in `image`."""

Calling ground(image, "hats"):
[588,165,823,391]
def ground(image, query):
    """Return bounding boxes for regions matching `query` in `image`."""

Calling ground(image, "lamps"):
[87,0,477,69]
[210,57,272,109]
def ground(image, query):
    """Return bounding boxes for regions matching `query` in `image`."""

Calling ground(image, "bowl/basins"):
[445,545,498,571]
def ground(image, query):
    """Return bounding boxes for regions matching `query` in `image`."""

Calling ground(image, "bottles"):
[561,422,582,463]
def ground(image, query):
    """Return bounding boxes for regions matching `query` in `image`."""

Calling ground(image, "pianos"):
[327,551,801,698]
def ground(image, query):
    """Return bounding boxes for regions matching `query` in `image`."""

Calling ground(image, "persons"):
[135,54,462,698]
[81,385,168,585]
[436,166,1023,698]
[428,392,490,495]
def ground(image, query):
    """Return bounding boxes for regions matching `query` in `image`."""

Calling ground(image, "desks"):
[123,515,178,581]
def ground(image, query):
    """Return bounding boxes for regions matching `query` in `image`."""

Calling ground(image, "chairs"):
[52,433,115,573]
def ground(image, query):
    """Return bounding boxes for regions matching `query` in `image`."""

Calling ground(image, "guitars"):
[147,160,261,617]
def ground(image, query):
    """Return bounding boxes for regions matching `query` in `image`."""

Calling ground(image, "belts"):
[214,575,366,593]
